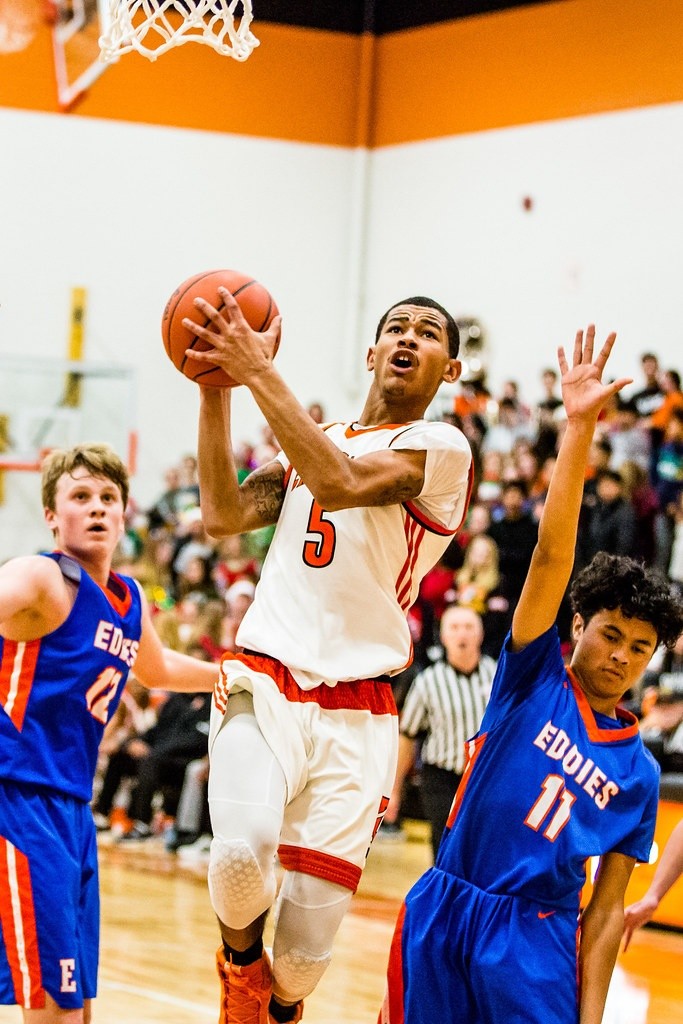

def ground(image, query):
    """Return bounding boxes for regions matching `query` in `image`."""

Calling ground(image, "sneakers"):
[265,992,304,1024]
[216,945,272,1024]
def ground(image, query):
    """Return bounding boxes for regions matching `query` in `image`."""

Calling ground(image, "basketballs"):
[160,268,282,390]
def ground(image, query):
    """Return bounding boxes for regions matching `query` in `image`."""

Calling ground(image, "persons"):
[0,444,224,1024]
[89,401,325,855]
[373,322,683,1023]
[181,286,474,1023]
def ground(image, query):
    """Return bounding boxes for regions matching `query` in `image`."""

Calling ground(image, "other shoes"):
[163,830,204,853]
[93,821,112,835]
[117,819,152,842]
